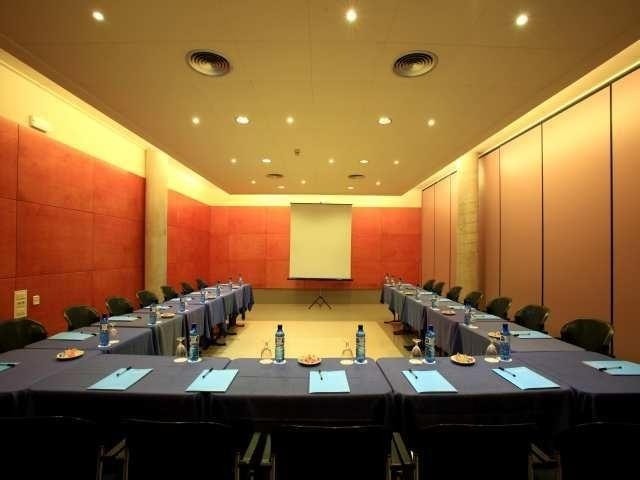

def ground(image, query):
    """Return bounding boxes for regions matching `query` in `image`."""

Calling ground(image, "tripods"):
[308,280,332,310]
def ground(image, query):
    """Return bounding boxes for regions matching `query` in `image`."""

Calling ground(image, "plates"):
[450,355,475,365]
[297,355,322,365]
[160,313,175,318]
[56,349,85,360]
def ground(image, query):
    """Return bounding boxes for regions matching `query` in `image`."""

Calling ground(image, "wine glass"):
[109,323,120,343]
[261,340,273,363]
[409,338,422,365]
[485,337,498,363]
[341,341,354,366]
[174,336,188,360]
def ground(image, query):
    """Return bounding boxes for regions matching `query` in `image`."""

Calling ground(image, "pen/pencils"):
[117,365,132,377]
[598,366,622,371]
[499,367,516,377]
[202,368,214,378]
[80,331,97,336]
[128,315,142,319]
[409,369,417,379]
[0,364,16,366]
[512,333,530,336]
[319,369,323,380]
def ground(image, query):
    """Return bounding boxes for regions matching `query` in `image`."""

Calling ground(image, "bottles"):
[100,313,109,347]
[355,324,365,363]
[179,295,186,312]
[424,325,436,363]
[149,301,156,324]
[383,271,440,311]
[463,299,471,325]
[224,272,243,295]
[276,324,284,361]
[189,324,200,359]
[200,285,206,303]
[501,324,513,362]
[216,279,221,296]
